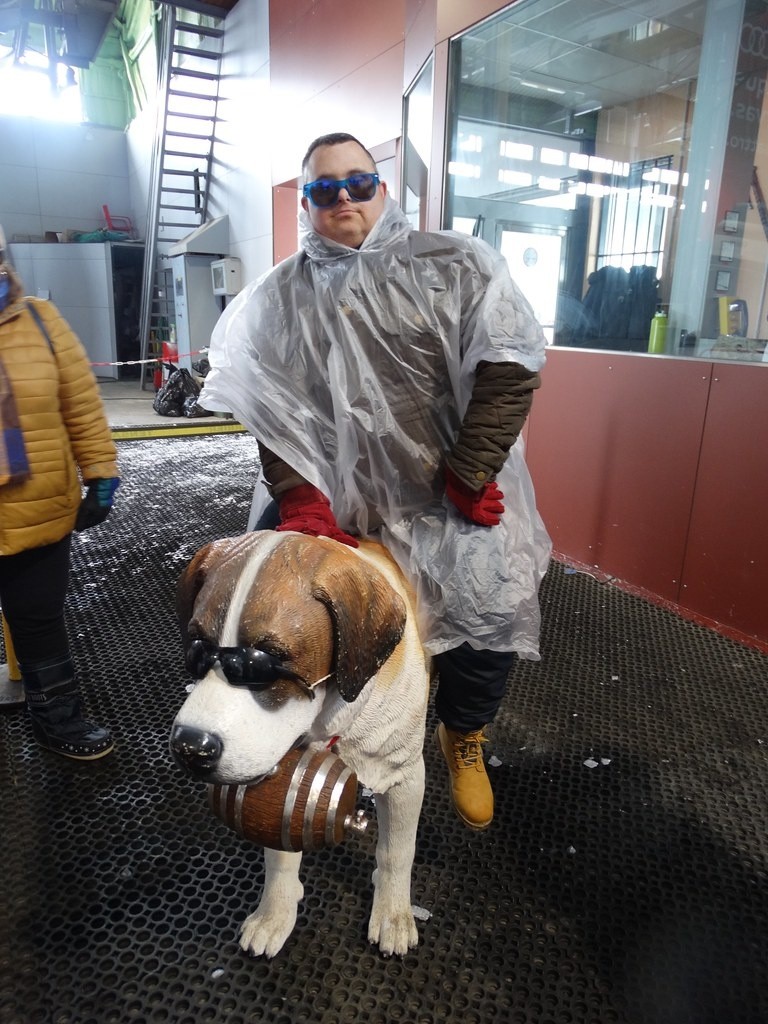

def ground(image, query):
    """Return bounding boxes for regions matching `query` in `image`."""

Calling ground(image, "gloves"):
[75,476,122,532]
[276,482,358,546]
[443,459,504,526]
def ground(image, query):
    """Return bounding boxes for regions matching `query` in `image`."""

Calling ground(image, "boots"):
[432,717,495,828]
[18,650,115,761]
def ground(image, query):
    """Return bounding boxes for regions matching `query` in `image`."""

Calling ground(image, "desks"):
[7,242,145,379]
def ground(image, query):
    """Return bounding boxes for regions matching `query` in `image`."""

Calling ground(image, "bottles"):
[648,310,667,355]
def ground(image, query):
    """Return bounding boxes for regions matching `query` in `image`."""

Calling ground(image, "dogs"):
[168,527,447,961]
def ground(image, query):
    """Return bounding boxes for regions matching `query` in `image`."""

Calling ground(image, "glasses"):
[303,172,380,209]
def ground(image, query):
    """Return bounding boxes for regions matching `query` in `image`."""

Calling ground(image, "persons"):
[244,131,542,828]
[0,225,124,763]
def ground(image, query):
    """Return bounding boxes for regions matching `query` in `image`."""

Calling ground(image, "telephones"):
[719,296,749,337]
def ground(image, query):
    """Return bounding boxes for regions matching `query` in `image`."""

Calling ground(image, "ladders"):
[140,2,223,390]
